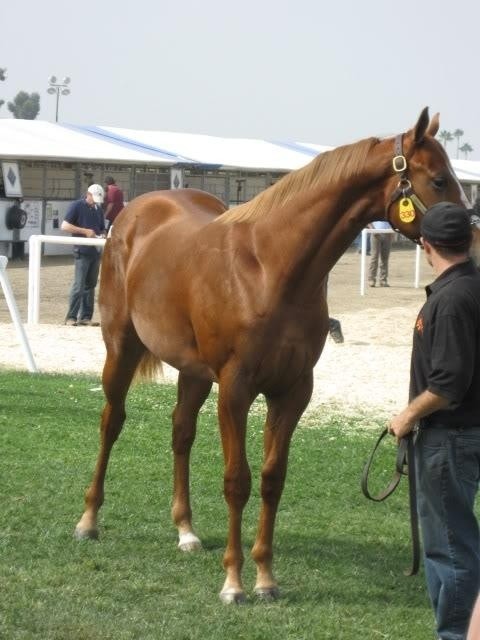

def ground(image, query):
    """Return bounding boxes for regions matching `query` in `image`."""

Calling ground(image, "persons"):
[103,176,124,231]
[385,203,480,639]
[357,226,372,256]
[367,220,396,287]
[63,183,99,326]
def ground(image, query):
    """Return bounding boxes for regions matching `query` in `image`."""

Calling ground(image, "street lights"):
[46,76,73,123]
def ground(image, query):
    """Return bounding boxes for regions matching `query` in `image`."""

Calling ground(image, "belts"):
[417,420,463,430]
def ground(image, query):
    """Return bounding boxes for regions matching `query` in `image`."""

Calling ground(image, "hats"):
[104,176,113,184]
[421,200,473,250]
[87,184,105,204]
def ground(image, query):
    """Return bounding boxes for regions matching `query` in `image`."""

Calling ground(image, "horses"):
[71,106,480,606]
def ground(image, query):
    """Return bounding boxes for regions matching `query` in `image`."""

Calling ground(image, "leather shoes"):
[368,280,377,287]
[380,283,390,287]
[79,318,100,327]
[65,318,77,326]
